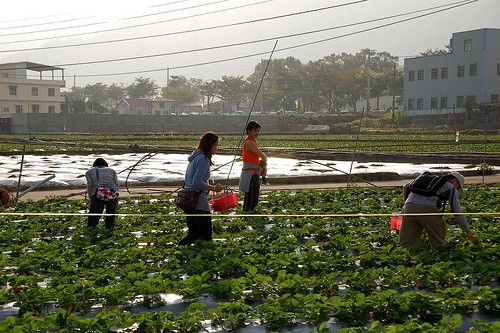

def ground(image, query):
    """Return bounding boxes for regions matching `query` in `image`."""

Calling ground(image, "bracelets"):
[213,186,215,190]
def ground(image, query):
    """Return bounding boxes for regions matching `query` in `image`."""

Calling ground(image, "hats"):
[450,172,464,191]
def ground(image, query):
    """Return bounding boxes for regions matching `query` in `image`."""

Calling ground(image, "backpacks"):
[409,171,447,196]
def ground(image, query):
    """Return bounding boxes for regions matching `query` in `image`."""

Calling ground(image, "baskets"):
[210,189,238,211]
[390,217,402,230]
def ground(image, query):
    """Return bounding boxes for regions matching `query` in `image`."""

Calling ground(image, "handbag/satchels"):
[175,191,200,209]
[96,186,119,201]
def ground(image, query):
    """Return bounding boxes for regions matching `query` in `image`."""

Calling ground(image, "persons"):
[239,120,267,214]
[398,171,473,250]
[259,160,270,186]
[177,131,218,247]
[85,158,120,230]
